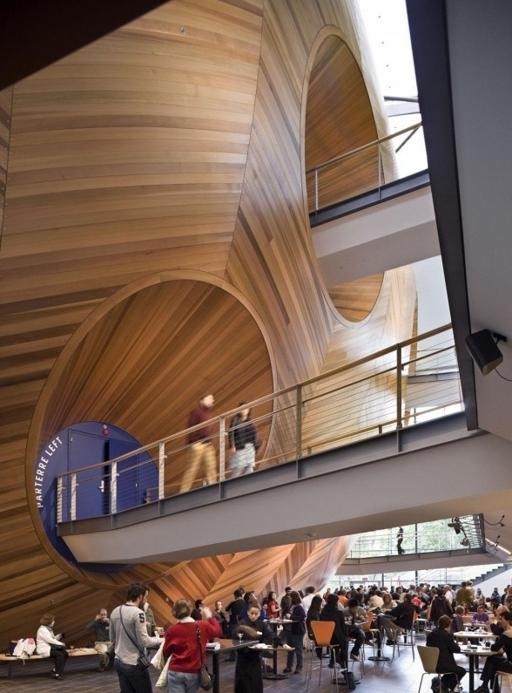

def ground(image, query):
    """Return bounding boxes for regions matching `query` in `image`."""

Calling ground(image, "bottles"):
[467,641,471,649]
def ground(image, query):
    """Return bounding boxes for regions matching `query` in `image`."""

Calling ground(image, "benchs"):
[0,648,98,679]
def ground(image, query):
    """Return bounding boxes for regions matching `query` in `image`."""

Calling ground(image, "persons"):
[177,394,218,493]
[228,401,261,479]
[86,584,512,692]
[36,613,69,679]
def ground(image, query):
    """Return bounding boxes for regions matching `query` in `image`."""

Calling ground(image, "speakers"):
[465,328,505,384]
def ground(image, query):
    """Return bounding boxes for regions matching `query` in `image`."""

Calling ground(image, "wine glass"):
[236,632,245,643]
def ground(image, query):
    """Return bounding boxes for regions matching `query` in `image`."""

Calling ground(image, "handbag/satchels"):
[137,656,152,670]
[5,641,23,657]
[199,663,216,691]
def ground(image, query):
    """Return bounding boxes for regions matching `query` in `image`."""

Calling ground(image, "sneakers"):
[364,641,375,646]
[282,666,292,673]
[386,639,398,645]
[55,675,64,680]
[292,670,303,675]
[436,680,454,692]
[98,666,105,673]
[327,656,348,669]
[349,652,362,663]
[473,686,489,693]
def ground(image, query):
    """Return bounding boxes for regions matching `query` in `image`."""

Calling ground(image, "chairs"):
[306,621,349,693]
[363,618,385,666]
[415,607,431,632]
[391,610,415,663]
[492,671,512,693]
[417,645,461,693]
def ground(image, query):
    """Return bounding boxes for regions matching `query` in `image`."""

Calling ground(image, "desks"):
[459,645,498,693]
[453,631,499,673]
[345,621,370,679]
[463,623,486,629]
[146,638,260,693]
[263,620,298,680]
